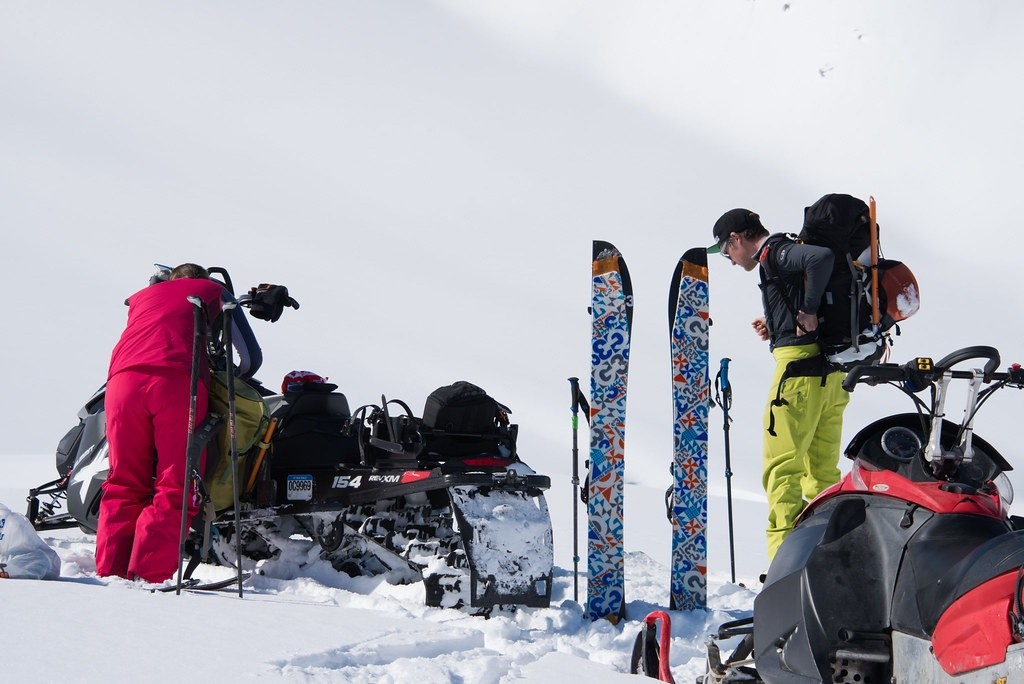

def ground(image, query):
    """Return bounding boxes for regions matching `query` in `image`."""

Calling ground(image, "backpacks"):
[189,370,271,511]
[770,193,920,355]
[422,380,512,456]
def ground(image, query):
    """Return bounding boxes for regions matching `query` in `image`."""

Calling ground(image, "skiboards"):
[149,568,253,593]
[586,239,638,626]
[666,246,713,615]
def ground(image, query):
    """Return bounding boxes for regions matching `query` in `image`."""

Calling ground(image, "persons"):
[95,264,263,584]
[713,208,852,564]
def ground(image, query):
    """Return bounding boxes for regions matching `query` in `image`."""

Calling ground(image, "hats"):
[705,208,759,253]
[282,371,339,393]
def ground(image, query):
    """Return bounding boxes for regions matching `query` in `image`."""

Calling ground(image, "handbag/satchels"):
[0,503,61,581]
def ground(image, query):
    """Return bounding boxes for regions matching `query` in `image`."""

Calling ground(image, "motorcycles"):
[25,282,555,621]
[629,346,1023,684]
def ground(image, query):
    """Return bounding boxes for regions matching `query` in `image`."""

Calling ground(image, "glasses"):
[719,239,732,259]
